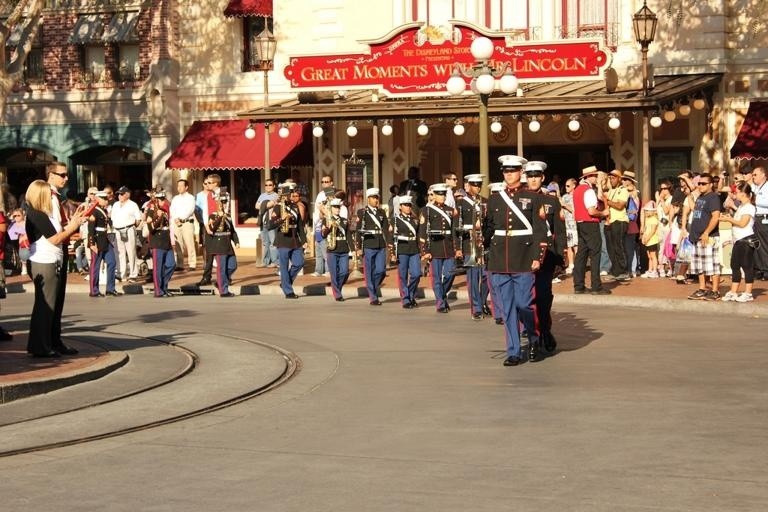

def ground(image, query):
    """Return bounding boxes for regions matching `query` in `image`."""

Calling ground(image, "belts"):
[398,236,416,241]
[115,225,132,230]
[218,232,231,236]
[289,224,298,228]
[463,224,474,230]
[336,236,346,240]
[157,226,169,231]
[432,230,452,235]
[494,229,531,238]
[95,227,106,232]
[370,230,382,235]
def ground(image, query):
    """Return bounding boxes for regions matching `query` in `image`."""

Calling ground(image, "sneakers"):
[196,280,211,286]
[267,262,280,268]
[175,267,184,271]
[311,272,320,277]
[45,349,58,357]
[220,292,234,297]
[59,342,78,355]
[592,287,611,295]
[322,271,330,276]
[688,288,753,302]
[575,285,591,294]
[600,265,686,284]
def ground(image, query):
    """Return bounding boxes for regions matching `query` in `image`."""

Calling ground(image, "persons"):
[483,154,565,366]
[546,165,768,302]
[255,166,508,322]
[2,172,240,299]
[25,163,92,359]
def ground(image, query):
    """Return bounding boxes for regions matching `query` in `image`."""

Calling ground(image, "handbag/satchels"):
[749,238,760,249]
[4,244,22,270]
[660,217,669,224]
[627,220,639,234]
[120,232,128,242]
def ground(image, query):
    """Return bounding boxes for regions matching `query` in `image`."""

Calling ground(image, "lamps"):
[278,122,289,139]
[244,122,255,139]
[679,96,691,115]
[692,93,704,110]
[650,111,662,127]
[382,121,393,136]
[664,102,676,122]
[568,116,580,131]
[418,120,428,136]
[346,122,357,137]
[490,117,501,132]
[453,119,465,135]
[312,122,323,138]
[608,113,620,129]
[529,116,541,133]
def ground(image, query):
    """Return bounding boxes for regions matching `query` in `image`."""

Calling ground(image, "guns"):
[361,211,368,249]
[459,196,464,251]
[394,213,398,260]
[472,194,485,321]
[426,204,432,253]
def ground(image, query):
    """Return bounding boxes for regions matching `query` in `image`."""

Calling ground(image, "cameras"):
[724,173,729,176]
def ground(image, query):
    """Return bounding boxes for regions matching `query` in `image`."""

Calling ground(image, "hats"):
[94,191,109,201]
[399,196,413,207]
[115,186,130,194]
[279,182,297,193]
[431,183,450,195]
[463,155,548,194]
[738,160,755,173]
[579,165,637,183]
[329,198,342,208]
[155,190,167,200]
[367,188,380,199]
[643,202,656,211]
[547,183,558,192]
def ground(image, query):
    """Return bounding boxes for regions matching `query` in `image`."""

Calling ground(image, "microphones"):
[85,197,91,211]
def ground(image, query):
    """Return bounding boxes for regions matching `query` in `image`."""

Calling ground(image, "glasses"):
[698,182,709,186]
[52,172,67,178]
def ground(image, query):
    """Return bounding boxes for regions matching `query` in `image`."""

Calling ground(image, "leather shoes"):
[437,308,448,313]
[95,292,105,297]
[163,291,172,297]
[336,298,344,301]
[472,304,556,366]
[370,300,381,305]
[106,290,122,296]
[286,293,298,298]
[403,300,418,308]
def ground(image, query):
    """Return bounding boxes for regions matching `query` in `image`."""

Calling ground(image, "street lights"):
[446,36,518,202]
[631,0,659,271]
[254,16,278,268]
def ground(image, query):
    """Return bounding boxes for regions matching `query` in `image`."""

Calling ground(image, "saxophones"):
[151,189,167,229]
[323,195,340,250]
[277,184,291,235]
[213,194,227,231]
